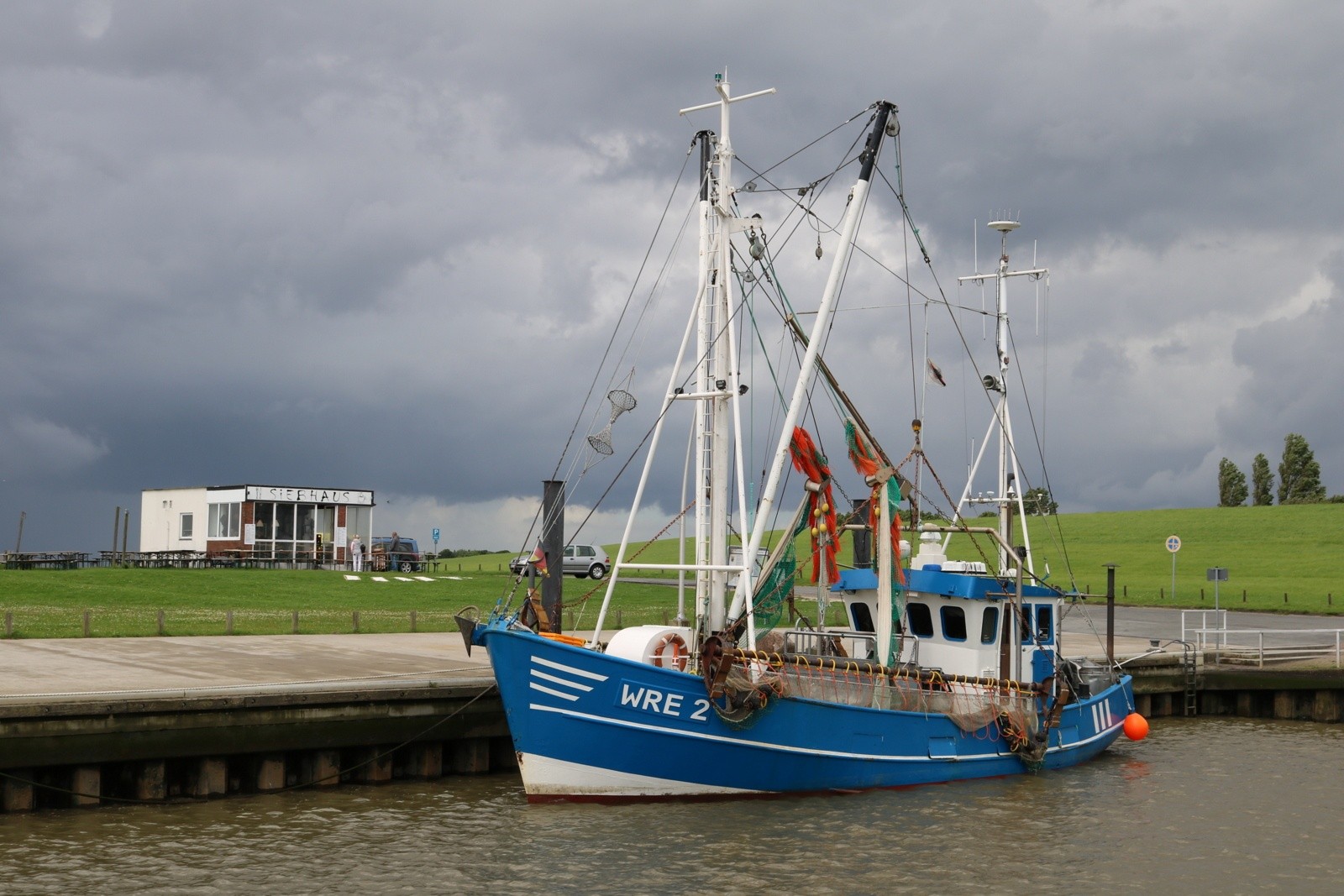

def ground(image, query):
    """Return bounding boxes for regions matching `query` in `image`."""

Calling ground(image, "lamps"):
[674,388,684,394]
[716,380,726,390]
[739,385,749,395]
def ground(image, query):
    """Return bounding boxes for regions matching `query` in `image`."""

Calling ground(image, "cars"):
[362,542,419,574]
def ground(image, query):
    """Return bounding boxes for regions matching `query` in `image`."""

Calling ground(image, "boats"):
[449,67,1150,810]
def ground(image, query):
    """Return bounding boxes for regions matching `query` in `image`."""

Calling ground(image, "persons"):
[389,532,399,571]
[350,535,362,572]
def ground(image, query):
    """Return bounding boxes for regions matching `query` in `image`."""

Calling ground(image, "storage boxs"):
[538,633,585,647]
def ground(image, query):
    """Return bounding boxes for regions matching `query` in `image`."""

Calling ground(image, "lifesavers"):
[653,633,688,672]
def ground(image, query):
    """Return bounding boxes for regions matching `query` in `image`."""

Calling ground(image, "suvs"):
[509,543,611,581]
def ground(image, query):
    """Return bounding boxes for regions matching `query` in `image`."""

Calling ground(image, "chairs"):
[565,549,574,556]
